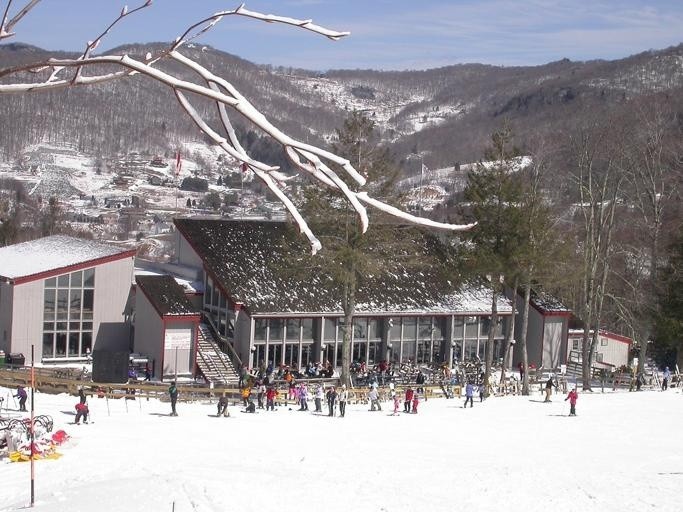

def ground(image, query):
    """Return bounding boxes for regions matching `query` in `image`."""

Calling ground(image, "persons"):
[217,397,229,416]
[240,359,483,417]
[169,382,178,416]
[12,386,28,410]
[544,378,557,402]
[518,360,524,380]
[662,369,668,391]
[564,388,578,416]
[75,403,89,424]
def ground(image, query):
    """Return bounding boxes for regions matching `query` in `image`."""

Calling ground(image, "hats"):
[342,384,346,388]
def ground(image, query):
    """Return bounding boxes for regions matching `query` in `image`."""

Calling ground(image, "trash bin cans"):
[6,353,25,368]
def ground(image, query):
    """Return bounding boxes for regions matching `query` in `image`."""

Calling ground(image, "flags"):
[174,152,181,177]
[239,162,249,173]
